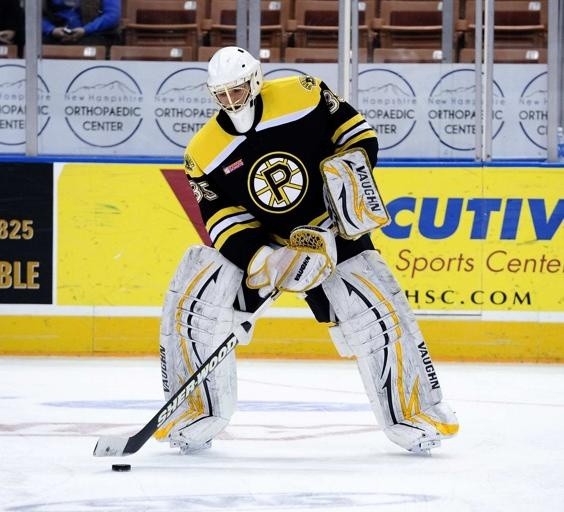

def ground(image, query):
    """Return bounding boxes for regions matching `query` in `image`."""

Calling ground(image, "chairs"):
[367,1,460,63]
[197,0,290,64]
[458,2,548,63]
[40,43,107,60]
[283,2,374,63]
[110,3,206,62]
[1,44,19,60]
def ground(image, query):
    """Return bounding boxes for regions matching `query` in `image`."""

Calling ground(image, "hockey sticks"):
[92,227,339,457]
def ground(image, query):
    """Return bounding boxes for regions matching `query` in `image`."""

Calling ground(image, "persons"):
[156,43,460,448]
[0,1,25,60]
[39,0,122,46]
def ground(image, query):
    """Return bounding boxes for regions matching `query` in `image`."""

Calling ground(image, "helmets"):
[204,46,264,135]
